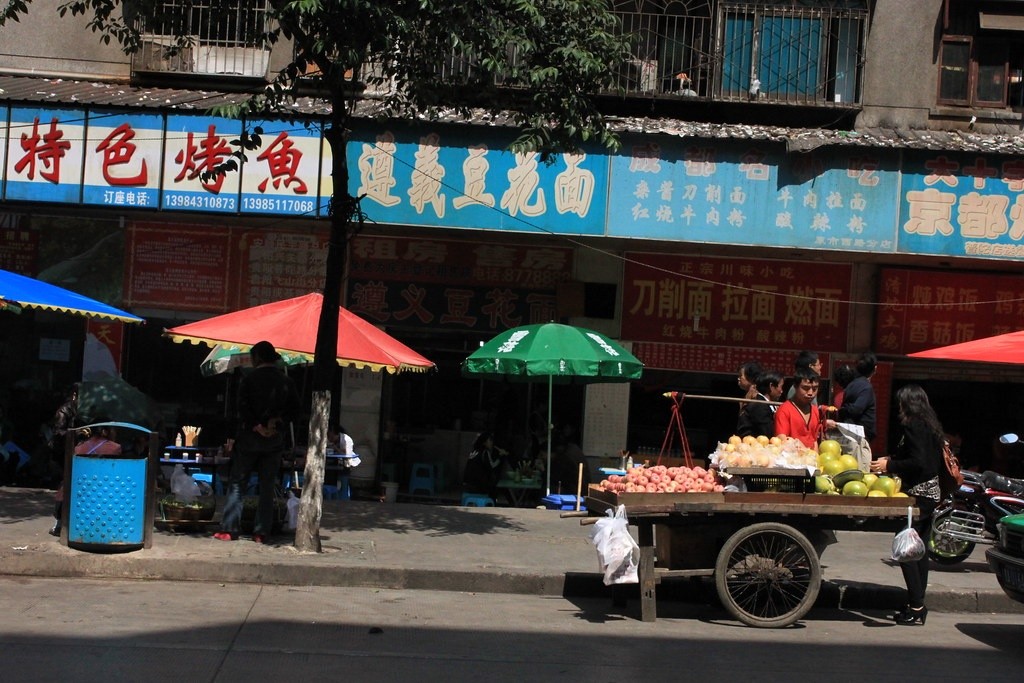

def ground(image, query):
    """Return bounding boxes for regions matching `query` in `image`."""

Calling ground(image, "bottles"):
[532,466,537,482]
[514,469,520,483]
[176,433,182,447]
[627,456,633,470]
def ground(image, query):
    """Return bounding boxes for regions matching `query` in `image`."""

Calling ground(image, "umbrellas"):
[906,330,1024,366]
[0,269,146,325]
[161,291,435,487]
[460,320,644,498]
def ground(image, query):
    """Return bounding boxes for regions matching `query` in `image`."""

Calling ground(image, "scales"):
[832,469,882,488]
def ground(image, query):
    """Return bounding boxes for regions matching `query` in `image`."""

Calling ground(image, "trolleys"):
[560,481,924,627]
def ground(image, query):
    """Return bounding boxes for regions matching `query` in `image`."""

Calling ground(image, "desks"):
[158,446,359,500]
[628,455,705,468]
[495,475,544,508]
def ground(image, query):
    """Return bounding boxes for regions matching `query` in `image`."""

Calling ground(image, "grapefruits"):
[812,440,910,498]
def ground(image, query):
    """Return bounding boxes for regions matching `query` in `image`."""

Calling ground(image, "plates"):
[831,469,864,488]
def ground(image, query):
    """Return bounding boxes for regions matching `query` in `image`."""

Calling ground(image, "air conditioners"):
[614,58,657,92]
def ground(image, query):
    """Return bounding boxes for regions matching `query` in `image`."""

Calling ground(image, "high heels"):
[894,606,929,625]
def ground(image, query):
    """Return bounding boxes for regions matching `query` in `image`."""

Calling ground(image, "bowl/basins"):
[522,478,533,483]
[506,470,515,479]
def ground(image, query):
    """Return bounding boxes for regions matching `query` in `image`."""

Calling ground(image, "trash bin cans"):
[59,421,158,546]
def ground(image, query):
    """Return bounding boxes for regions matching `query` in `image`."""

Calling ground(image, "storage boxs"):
[741,470,816,493]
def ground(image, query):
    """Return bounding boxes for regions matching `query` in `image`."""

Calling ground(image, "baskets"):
[724,467,810,492]
[162,501,216,533]
[238,483,287,535]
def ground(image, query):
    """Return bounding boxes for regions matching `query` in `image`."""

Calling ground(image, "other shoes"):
[211,530,232,540]
[253,535,272,543]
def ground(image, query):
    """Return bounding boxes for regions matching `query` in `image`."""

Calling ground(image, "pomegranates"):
[719,435,821,468]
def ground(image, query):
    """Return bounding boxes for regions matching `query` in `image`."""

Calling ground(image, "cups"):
[164,453,170,460]
[196,454,202,463]
[183,453,188,460]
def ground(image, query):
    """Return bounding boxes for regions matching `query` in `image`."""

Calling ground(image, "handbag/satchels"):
[937,440,964,504]
[890,506,925,563]
[281,490,299,534]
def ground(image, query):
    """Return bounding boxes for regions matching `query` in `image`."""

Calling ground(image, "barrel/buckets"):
[380,482,399,503]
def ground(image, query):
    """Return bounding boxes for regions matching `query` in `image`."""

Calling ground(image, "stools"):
[409,464,439,495]
[461,492,495,507]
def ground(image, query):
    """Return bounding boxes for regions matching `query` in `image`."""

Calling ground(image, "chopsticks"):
[493,446,508,455]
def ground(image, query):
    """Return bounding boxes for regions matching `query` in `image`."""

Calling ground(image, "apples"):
[598,465,727,493]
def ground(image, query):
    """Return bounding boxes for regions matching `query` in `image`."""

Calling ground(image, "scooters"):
[928,434,1023,563]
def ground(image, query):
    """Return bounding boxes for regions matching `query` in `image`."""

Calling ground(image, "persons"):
[832,364,855,417]
[736,349,836,449]
[870,384,945,625]
[466,432,509,505]
[49,383,164,537]
[942,423,988,471]
[513,415,580,494]
[838,352,878,442]
[327,421,361,477]
[207,341,302,543]
